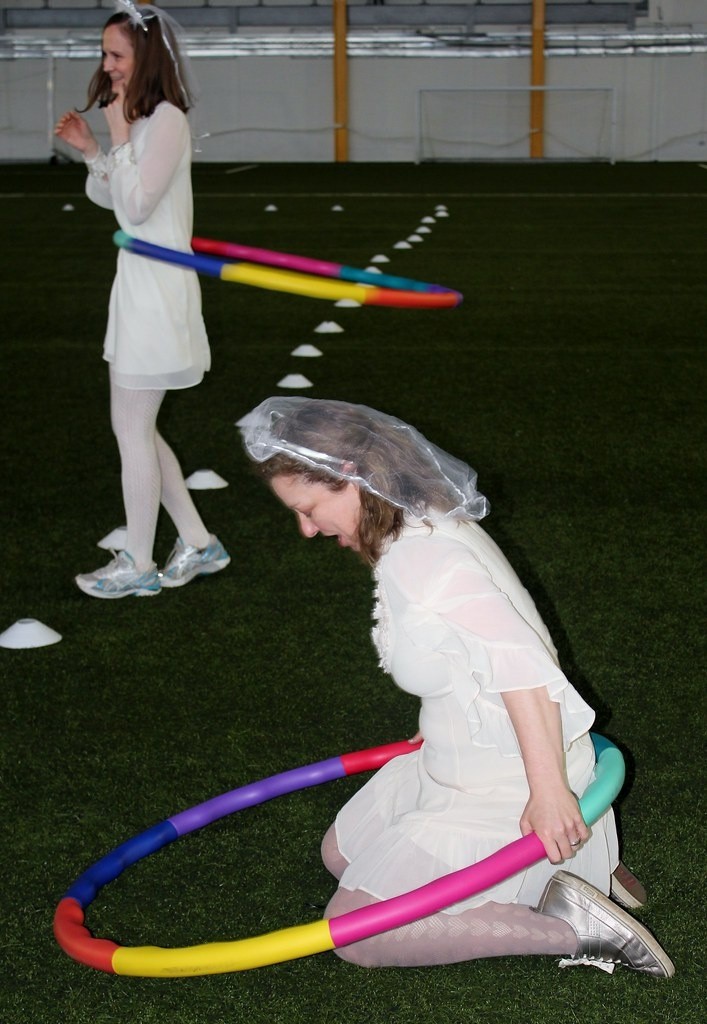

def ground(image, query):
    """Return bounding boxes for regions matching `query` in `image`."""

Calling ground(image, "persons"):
[53,0,233,599]
[239,396,682,978]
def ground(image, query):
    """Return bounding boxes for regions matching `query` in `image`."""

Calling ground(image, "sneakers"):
[74,551,162,599]
[161,535,232,589]
[535,862,676,979]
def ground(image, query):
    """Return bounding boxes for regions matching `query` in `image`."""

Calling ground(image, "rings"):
[570,837,581,846]
